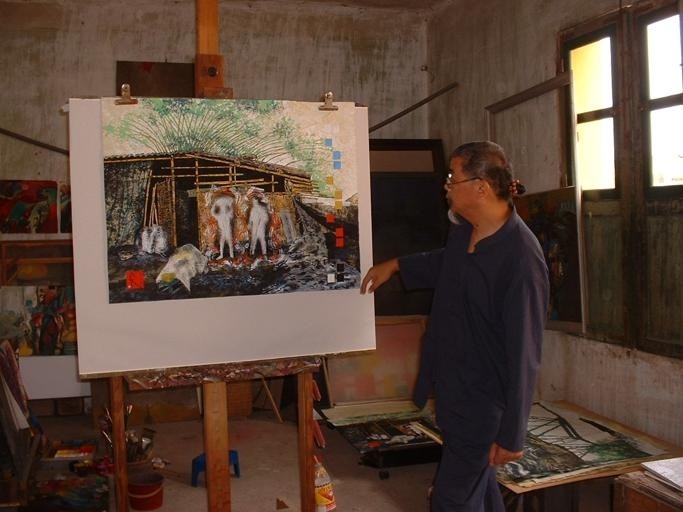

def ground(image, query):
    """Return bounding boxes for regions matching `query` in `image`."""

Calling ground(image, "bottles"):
[313,460,335,511]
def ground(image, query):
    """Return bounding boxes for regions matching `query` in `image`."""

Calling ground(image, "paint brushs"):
[102,403,159,461]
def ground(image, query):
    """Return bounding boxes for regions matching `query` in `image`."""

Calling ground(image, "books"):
[640,457,683,492]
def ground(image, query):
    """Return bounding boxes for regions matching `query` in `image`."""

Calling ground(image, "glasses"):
[445,173,482,188]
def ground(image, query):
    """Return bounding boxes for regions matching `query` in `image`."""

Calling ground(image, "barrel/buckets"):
[127,471,165,511]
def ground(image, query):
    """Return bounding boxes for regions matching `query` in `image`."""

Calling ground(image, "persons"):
[360,140,551,512]
